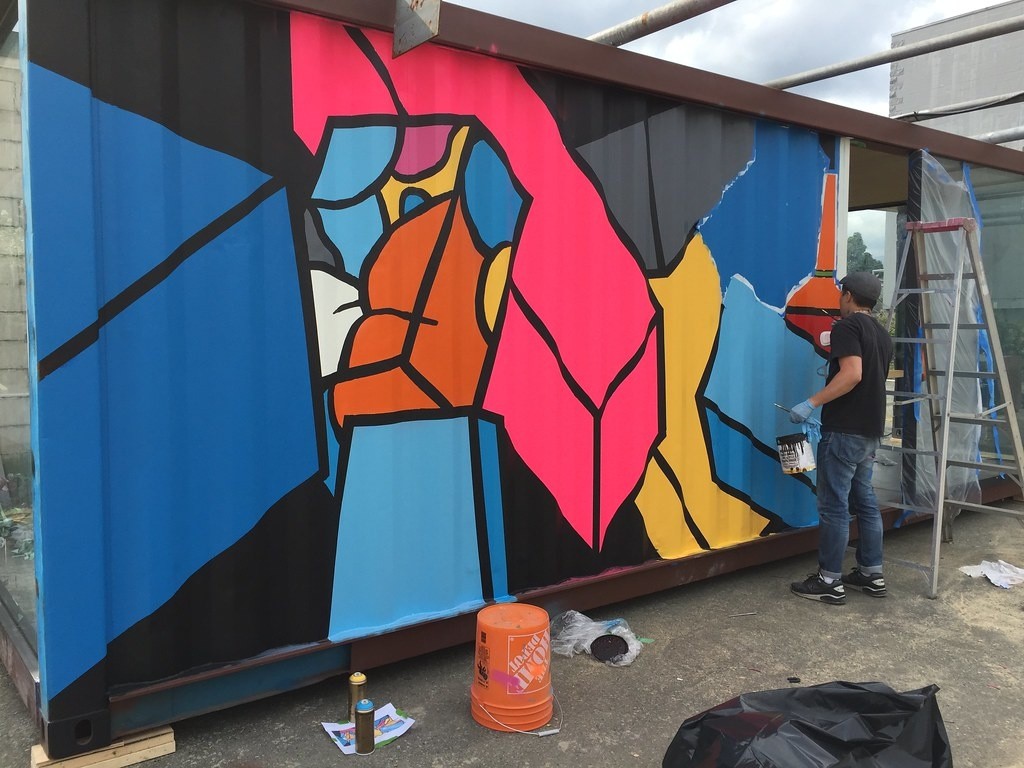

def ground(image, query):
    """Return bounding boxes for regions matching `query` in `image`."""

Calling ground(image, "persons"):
[790,272,894,604]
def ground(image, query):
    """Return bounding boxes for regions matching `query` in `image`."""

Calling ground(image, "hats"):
[839,271,881,301]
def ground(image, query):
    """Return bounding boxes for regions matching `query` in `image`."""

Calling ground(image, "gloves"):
[789,399,815,424]
[801,417,823,443]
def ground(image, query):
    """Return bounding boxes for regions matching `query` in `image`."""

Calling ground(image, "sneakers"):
[790,571,848,605]
[841,567,887,598]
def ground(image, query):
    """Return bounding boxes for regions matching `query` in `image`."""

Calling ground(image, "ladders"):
[845,217,1024,600]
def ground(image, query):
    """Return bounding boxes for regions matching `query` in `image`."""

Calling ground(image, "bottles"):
[355,699,376,756]
[347,672,367,723]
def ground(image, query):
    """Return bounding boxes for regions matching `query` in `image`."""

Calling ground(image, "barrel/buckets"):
[774,418,816,473]
[470,602,563,738]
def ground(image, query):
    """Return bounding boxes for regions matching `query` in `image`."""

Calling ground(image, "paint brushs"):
[773,402,791,413]
[820,307,840,323]
